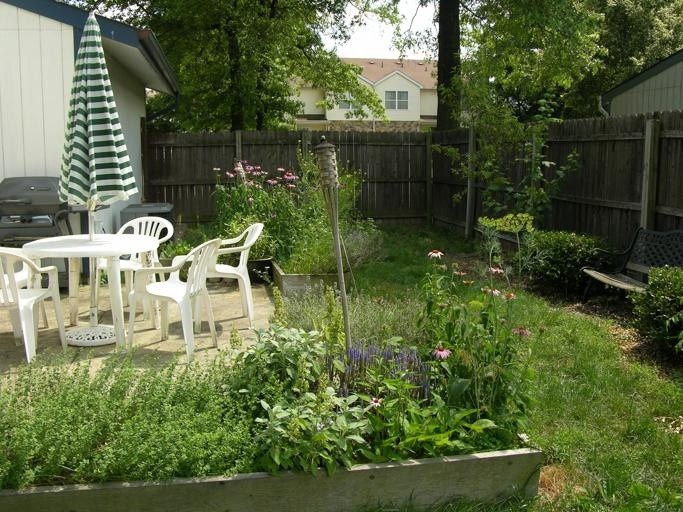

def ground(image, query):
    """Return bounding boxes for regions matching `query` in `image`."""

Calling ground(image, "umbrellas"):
[57,8,140,346]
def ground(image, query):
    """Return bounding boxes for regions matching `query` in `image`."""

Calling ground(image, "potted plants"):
[228,212,279,284]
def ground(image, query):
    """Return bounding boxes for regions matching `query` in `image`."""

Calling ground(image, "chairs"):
[0,175,73,280]
[0,214,264,364]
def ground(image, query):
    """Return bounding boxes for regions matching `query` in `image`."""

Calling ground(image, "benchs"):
[576,226,682,305]
